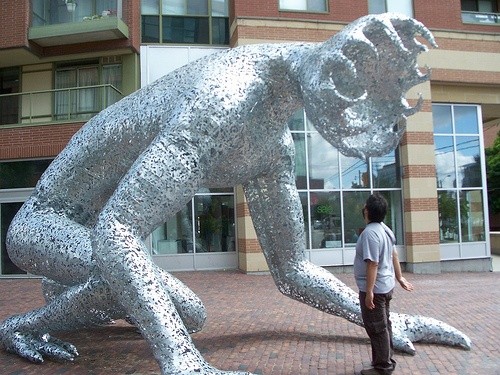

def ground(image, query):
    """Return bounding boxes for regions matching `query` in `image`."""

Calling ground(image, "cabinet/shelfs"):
[156,238,183,254]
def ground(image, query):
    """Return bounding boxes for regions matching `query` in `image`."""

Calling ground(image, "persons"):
[355,192,415,375]
[202,195,229,252]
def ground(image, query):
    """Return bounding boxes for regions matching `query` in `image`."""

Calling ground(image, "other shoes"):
[361,369,393,375]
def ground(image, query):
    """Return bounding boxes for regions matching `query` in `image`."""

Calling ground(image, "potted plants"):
[82,9,114,22]
[63,0,78,11]
